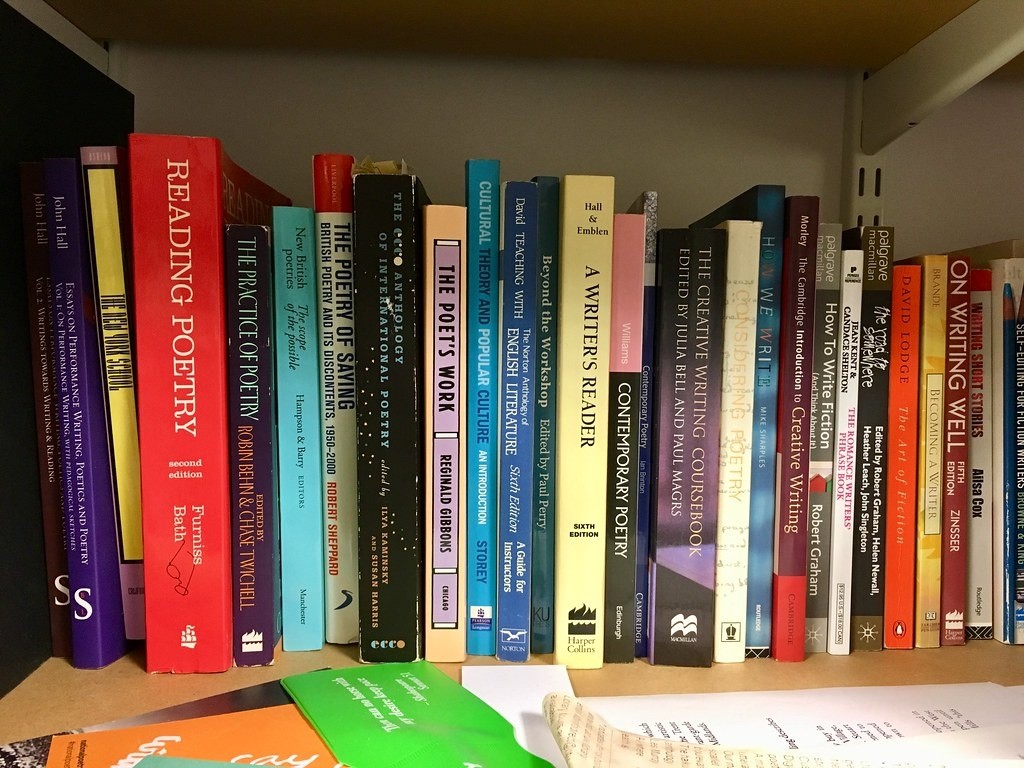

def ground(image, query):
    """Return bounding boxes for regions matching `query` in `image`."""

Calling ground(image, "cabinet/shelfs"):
[0,0,1024,768]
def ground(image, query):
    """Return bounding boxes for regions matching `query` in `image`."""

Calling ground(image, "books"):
[27,132,1024,674]
